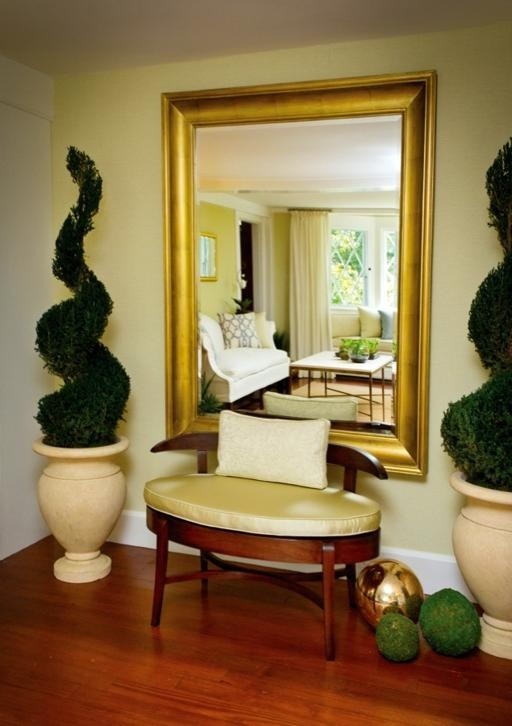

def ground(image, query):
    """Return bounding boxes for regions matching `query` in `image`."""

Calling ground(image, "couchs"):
[198,312,292,410]
[331,314,398,352]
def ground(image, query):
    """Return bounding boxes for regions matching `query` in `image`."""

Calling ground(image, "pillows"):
[216,312,260,348]
[211,409,332,489]
[358,305,381,337]
[378,309,399,340]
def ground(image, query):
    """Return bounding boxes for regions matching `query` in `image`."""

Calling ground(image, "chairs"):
[142,432,387,661]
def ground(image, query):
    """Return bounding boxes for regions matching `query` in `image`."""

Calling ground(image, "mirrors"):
[160,70,436,482]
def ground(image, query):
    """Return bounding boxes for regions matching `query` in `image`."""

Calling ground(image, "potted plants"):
[441,119,512,659]
[31,147,130,584]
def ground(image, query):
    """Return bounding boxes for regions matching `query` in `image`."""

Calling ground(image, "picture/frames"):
[198,232,217,282]
